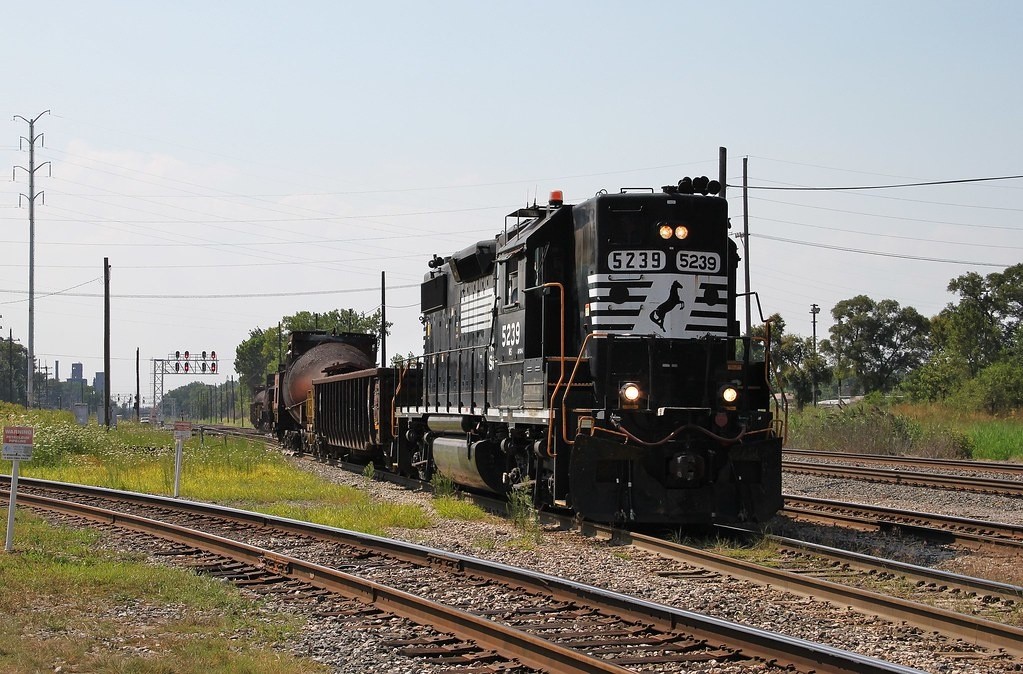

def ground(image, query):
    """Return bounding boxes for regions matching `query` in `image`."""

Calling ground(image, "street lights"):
[808,304,821,408]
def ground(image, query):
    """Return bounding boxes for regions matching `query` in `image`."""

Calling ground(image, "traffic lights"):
[185,363,188,371]
[185,351,189,358]
[211,351,215,358]
[212,363,216,371]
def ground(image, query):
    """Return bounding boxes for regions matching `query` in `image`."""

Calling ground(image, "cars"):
[140,417,150,423]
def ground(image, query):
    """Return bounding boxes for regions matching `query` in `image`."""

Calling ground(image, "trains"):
[249,175,785,529]
